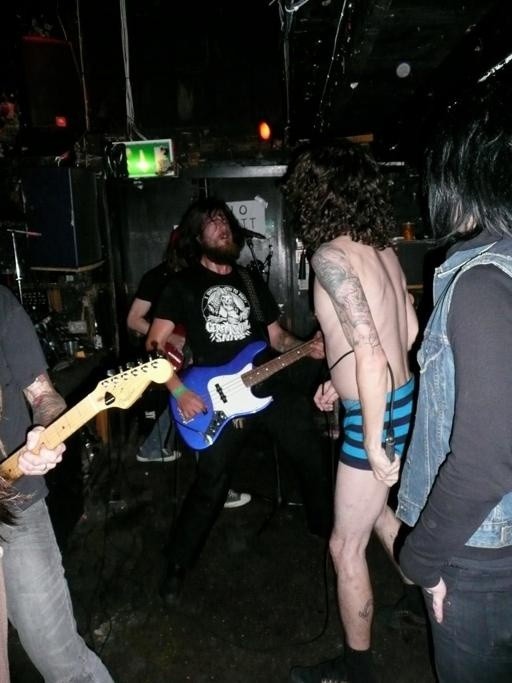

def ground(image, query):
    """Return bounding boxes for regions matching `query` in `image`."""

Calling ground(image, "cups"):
[401,222,416,241]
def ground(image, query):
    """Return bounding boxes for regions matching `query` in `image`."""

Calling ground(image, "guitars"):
[169,337,322,450]
[0,356,175,487]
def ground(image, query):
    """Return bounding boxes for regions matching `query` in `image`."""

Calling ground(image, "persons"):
[0,283,121,682]
[128,196,341,591]
[277,128,443,681]
[122,224,253,510]
[387,52,511,681]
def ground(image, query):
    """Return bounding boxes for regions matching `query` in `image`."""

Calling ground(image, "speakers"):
[293,247,328,343]
[18,162,105,267]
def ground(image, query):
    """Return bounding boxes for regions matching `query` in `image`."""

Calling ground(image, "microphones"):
[236,225,266,243]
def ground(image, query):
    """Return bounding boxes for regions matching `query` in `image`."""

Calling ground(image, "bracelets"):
[172,384,187,398]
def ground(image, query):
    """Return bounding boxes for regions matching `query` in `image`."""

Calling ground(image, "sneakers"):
[305,514,336,548]
[376,596,428,634]
[288,655,385,683]
[159,558,186,606]
[135,446,183,463]
[223,488,253,509]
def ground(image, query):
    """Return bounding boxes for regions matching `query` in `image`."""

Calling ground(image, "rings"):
[41,464,47,471]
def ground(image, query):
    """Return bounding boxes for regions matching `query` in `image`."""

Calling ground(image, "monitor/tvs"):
[111,139,179,181]
